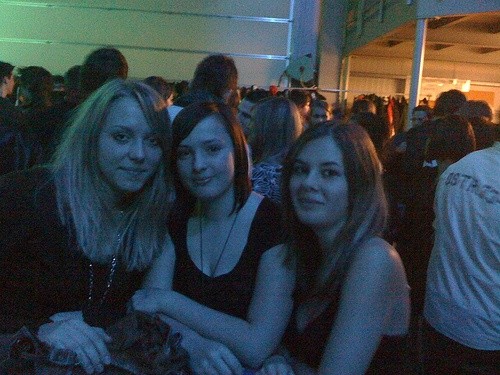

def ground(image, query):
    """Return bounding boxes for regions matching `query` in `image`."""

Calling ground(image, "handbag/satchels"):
[0,310,194,375]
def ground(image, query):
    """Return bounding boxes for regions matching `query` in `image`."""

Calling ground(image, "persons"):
[0,47,500,284]
[0,78,173,375]
[423,140,500,375]
[125,102,298,375]
[253,120,411,375]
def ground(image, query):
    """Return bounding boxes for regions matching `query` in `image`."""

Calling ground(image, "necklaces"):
[199,199,240,303]
[87,182,134,326]
